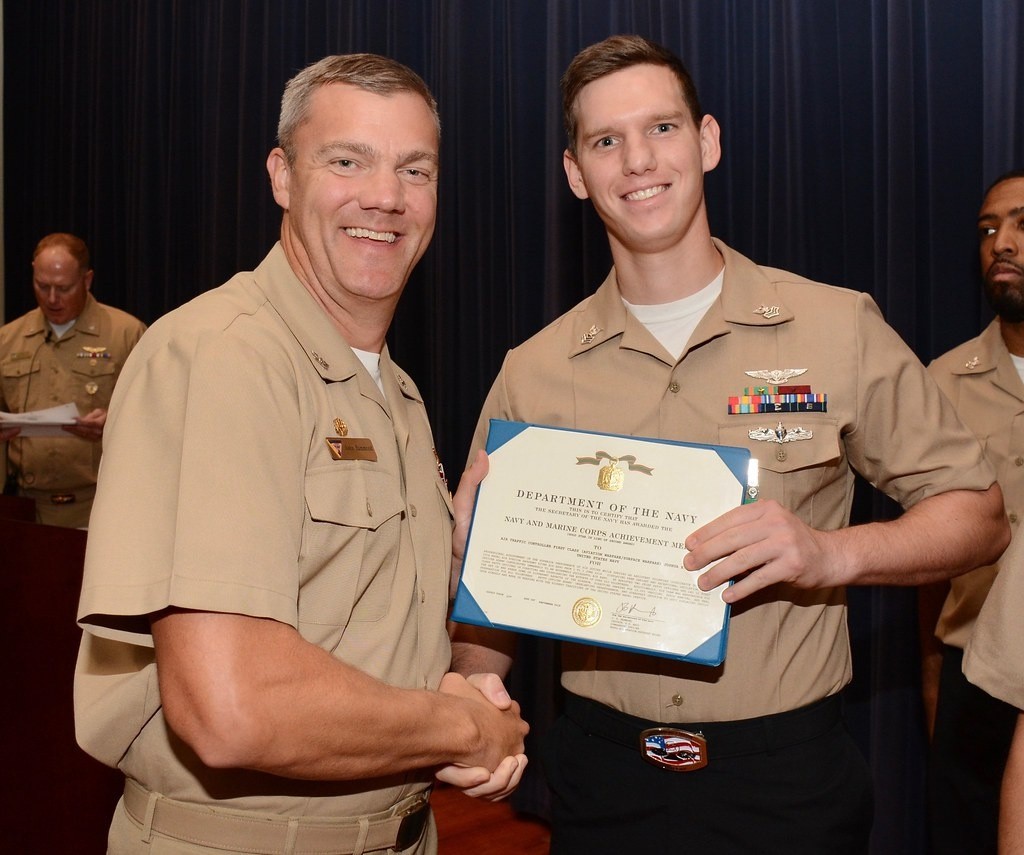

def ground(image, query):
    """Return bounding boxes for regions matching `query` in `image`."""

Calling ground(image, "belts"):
[121,778,428,852]
[561,692,840,770]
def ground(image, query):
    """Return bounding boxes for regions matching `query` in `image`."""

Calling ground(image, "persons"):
[73,55,530,855]
[0,233,148,531]
[437,36,1011,855]
[926,173,1024,855]
